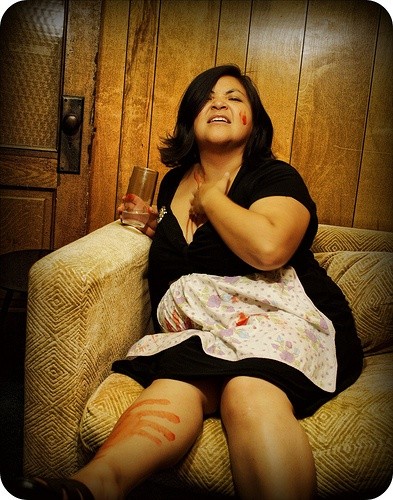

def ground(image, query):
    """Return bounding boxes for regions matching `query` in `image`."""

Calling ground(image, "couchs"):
[25,217,392,493]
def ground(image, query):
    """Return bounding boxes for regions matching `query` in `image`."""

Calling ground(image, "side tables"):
[0,250,53,328]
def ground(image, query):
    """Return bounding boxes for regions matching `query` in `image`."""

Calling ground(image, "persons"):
[24,63,362,500]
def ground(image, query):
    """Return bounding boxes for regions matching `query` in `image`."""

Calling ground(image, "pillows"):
[311,251,393,357]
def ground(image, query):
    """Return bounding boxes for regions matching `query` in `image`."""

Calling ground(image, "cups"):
[120,165,160,228]
